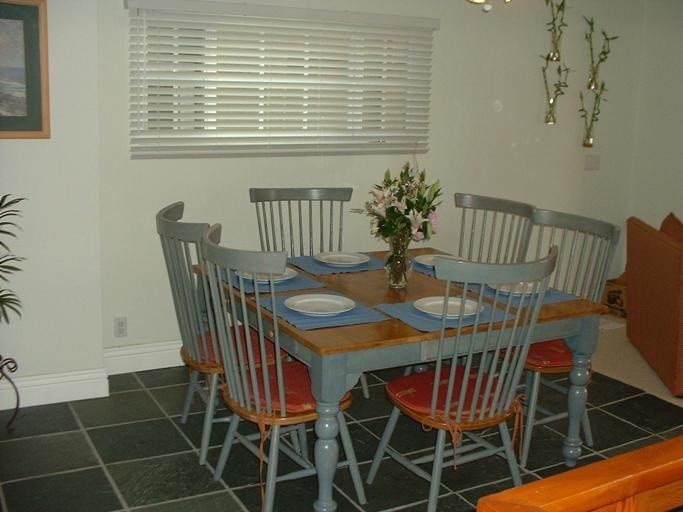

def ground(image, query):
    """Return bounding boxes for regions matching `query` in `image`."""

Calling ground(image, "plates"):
[312,250,370,268]
[487,281,549,297]
[234,266,297,283]
[412,294,485,320]
[283,293,357,317]
[414,254,468,269]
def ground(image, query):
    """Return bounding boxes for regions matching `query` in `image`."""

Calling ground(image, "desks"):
[190,246,609,512]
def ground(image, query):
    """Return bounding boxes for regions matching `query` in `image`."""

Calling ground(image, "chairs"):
[367,242,559,512]
[157,198,288,466]
[445,191,536,367]
[514,207,622,468]
[198,222,371,512]
[249,187,371,401]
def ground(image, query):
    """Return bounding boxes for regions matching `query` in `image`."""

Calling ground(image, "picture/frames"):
[0,0,51,140]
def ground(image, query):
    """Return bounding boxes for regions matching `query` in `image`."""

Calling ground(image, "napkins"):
[216,261,327,295]
[253,287,391,330]
[409,252,585,310]
[286,249,388,276]
[373,294,516,333]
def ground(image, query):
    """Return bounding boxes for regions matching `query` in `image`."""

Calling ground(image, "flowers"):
[348,161,448,282]
